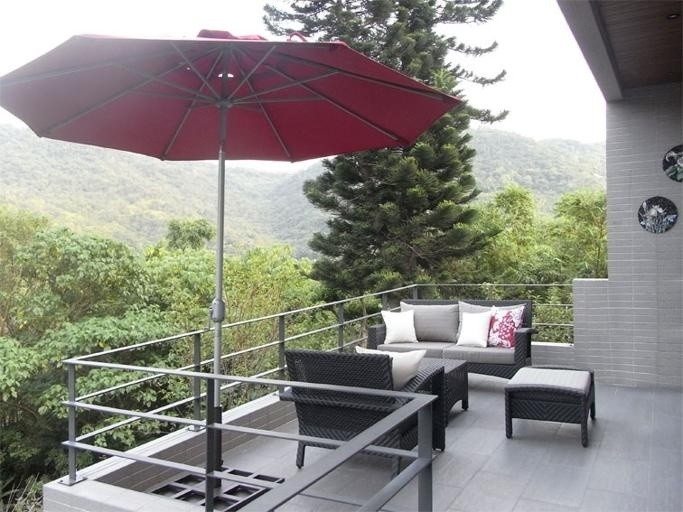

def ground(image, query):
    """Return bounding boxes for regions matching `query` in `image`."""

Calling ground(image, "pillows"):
[355,344,427,391]
[380,300,527,348]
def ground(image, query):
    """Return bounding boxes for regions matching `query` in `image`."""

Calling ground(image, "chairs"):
[277,345,444,481]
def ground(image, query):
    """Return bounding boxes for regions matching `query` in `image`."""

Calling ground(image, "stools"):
[504,366,597,448]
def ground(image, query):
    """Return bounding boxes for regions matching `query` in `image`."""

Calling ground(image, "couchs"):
[366,300,537,379]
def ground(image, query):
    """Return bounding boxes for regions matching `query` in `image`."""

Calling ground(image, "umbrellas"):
[0,28,465,489]
